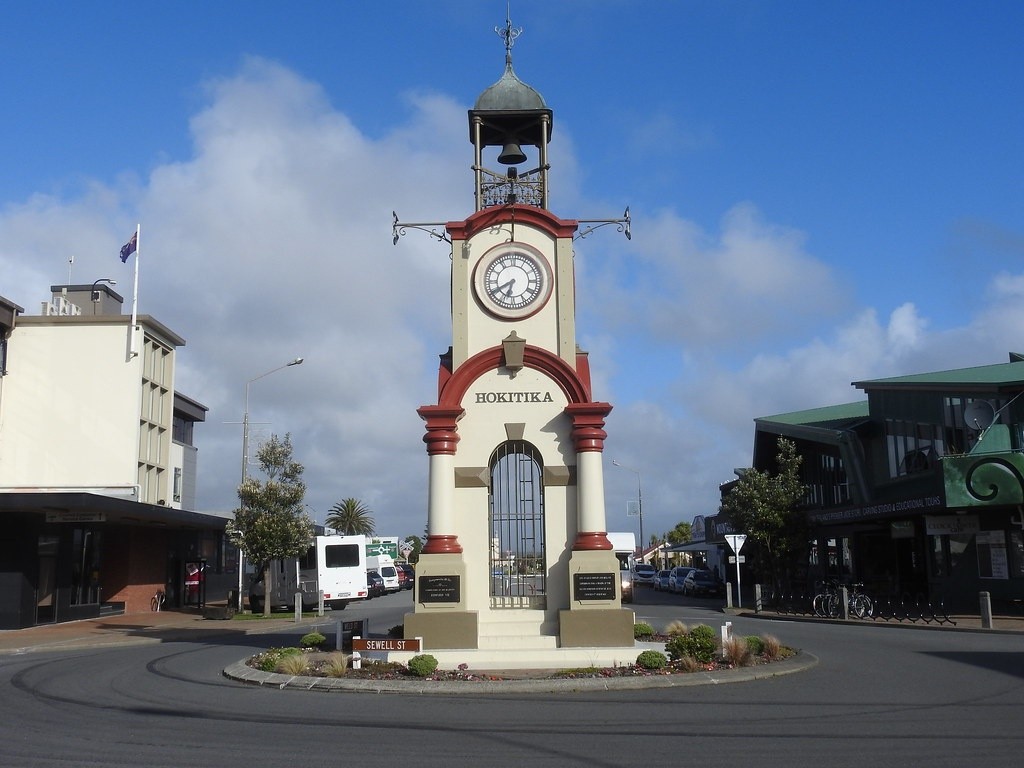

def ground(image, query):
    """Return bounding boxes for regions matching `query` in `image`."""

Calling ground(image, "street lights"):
[241,356,304,509]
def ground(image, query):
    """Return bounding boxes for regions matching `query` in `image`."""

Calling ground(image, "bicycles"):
[813,580,873,620]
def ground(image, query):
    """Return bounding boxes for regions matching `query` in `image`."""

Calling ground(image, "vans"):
[366,553,399,595]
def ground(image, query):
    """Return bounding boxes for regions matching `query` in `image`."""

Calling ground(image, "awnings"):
[659,540,709,552]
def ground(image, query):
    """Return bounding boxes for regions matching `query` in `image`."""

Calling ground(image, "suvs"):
[633,563,657,587]
[395,564,415,591]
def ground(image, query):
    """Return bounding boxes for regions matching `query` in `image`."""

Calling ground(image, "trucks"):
[248,534,369,613]
[365,570,386,601]
[605,532,636,603]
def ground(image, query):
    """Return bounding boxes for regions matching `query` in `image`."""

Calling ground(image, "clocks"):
[473,241,554,322]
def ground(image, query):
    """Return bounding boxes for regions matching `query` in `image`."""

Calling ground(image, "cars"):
[683,570,727,600]
[668,566,696,594]
[653,570,671,591]
